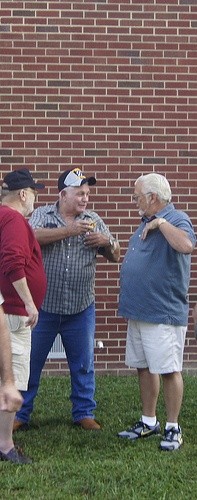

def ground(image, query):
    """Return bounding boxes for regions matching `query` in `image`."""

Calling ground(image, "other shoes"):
[73,418,100,429]
[0,447,32,465]
[13,419,29,432]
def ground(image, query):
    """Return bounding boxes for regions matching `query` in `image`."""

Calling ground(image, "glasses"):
[132,192,153,201]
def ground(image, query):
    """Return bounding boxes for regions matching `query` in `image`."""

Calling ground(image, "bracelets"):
[156,218,166,229]
[105,237,114,248]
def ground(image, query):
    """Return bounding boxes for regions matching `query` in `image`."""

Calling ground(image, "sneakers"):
[117,420,161,439]
[159,424,183,450]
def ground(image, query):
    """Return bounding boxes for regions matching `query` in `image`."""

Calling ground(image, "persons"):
[0,170,47,464]
[119,173,196,451]
[0,291,24,413]
[11,168,120,433]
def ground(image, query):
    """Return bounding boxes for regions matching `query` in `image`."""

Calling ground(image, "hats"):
[57,168,96,191]
[1,168,44,191]
